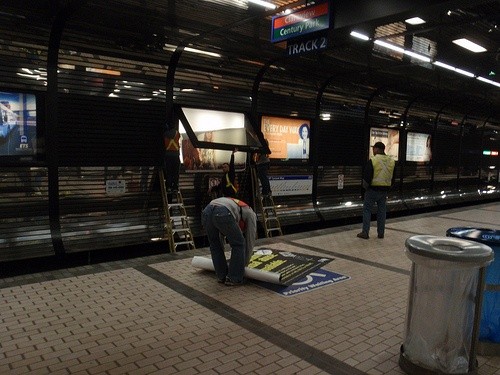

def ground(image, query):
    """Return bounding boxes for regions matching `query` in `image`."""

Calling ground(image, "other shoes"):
[224,277,244,285]
[218,276,225,283]
[357,231,369,239]
[378,233,384,239]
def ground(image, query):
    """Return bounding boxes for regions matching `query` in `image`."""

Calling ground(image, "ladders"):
[257,173,284,238]
[158,167,197,254]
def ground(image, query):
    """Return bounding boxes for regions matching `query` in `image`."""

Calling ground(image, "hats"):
[371,142,385,150]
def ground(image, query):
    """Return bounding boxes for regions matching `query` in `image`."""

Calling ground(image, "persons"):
[201,197,257,287]
[299,123,310,158]
[220,147,238,197]
[164,121,180,203]
[357,141,396,239]
[246,128,271,195]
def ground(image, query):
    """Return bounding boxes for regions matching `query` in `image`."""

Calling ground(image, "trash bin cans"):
[397,234,494,375]
[444,227,500,357]
